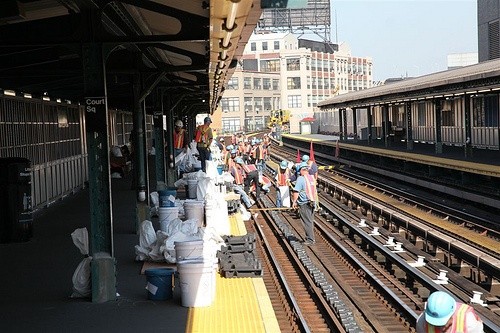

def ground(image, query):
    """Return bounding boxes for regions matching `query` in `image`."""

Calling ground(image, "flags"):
[297,142,338,164]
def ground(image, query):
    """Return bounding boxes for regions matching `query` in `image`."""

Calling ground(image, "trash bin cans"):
[300,121,319,135]
[111,168,137,234]
[0,157,33,244]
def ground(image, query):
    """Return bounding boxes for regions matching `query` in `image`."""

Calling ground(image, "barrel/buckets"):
[176,258,218,308]
[157,155,235,274]
[144,268,175,303]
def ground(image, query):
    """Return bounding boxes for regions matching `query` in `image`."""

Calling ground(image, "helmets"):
[302,155,309,163]
[226,146,231,150]
[425,291,457,326]
[300,162,309,169]
[237,159,242,164]
[232,132,262,146]
[280,161,287,169]
[296,165,301,171]
[220,138,224,141]
[231,150,236,154]
[175,120,183,126]
[204,117,212,123]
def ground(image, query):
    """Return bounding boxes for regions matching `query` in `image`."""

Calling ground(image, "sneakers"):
[302,239,313,244]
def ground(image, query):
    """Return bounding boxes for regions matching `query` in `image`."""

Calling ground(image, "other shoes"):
[267,189,270,192]
[289,212,301,219]
[256,194,259,198]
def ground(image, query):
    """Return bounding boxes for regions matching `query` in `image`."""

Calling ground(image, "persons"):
[173,117,318,245]
[416,290,484,333]
